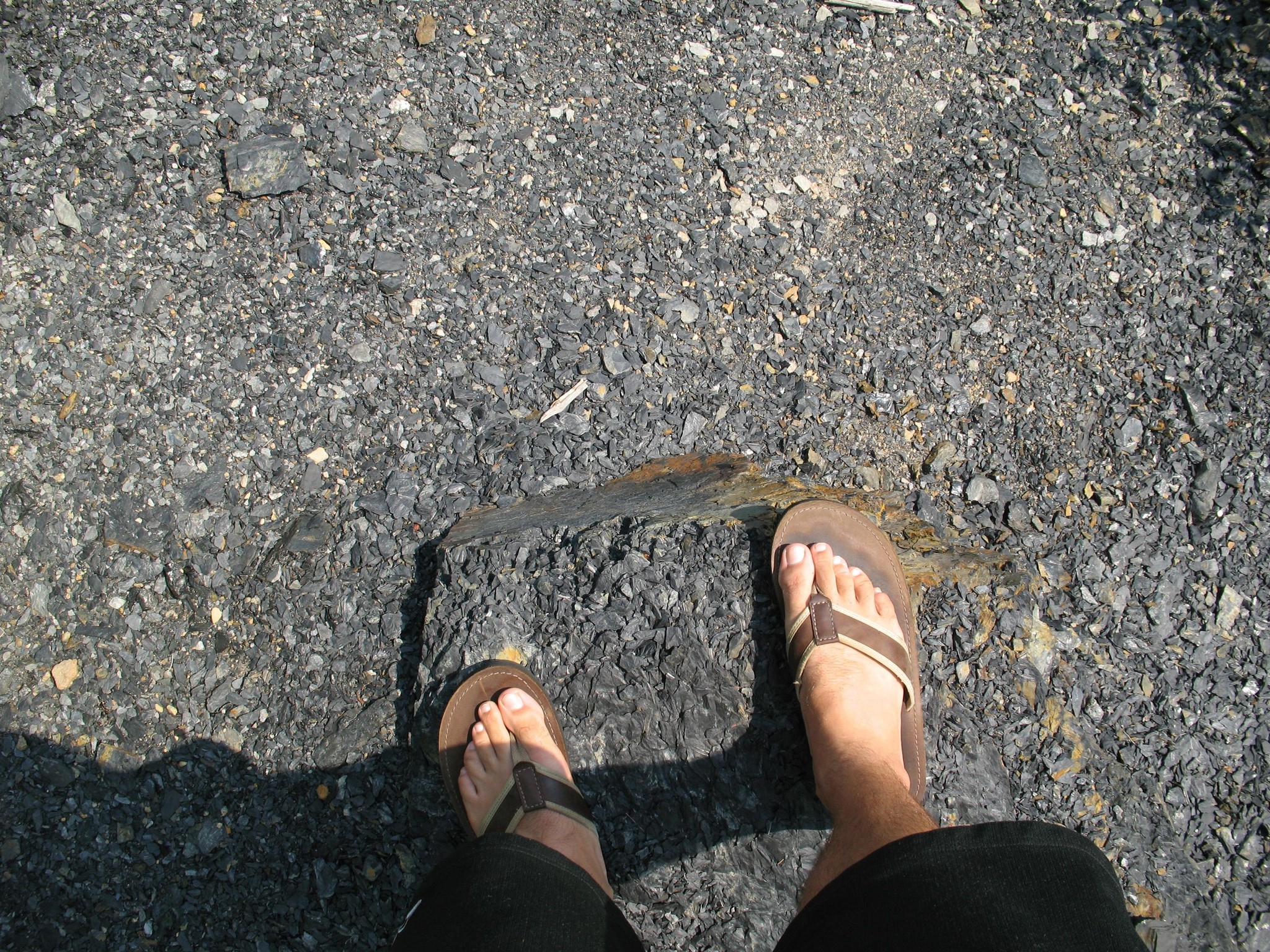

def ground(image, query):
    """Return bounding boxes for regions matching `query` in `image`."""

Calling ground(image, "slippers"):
[438,660,599,847]
[771,499,926,806]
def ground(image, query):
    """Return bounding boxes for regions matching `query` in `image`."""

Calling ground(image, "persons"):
[411,499,1147,952]
